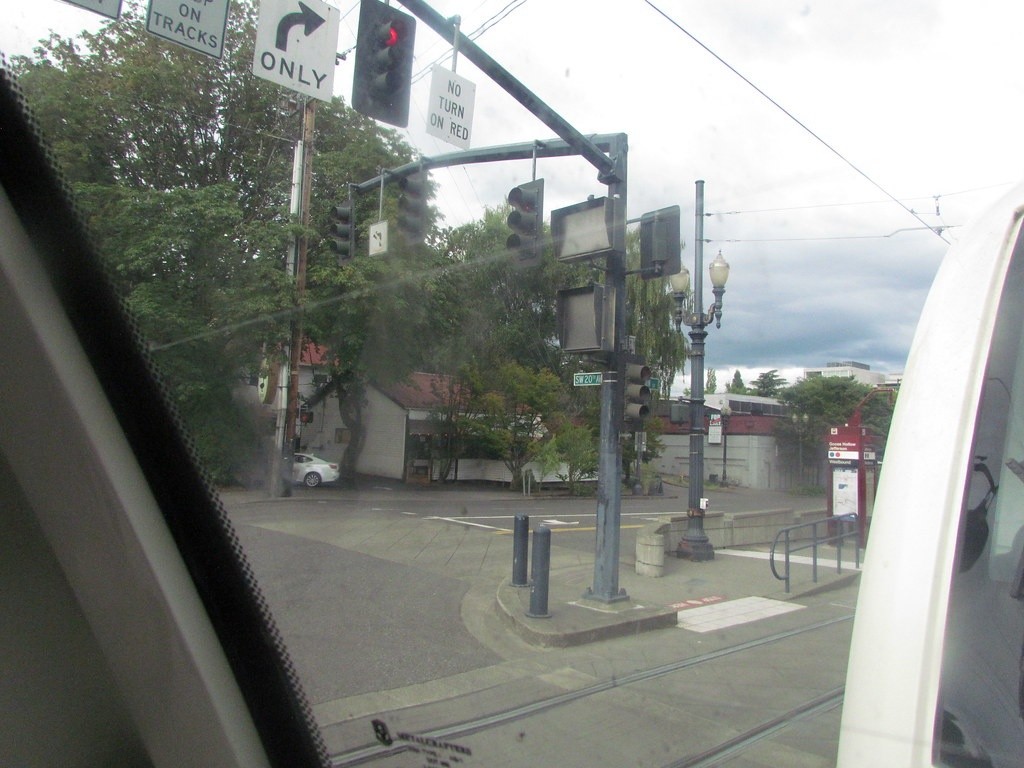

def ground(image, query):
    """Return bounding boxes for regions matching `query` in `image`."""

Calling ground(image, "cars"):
[292,453,339,487]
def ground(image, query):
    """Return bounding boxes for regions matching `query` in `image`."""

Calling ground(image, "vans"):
[832,180,1023,768]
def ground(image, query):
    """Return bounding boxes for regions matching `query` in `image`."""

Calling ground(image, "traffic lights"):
[506,179,545,266]
[557,284,617,355]
[351,1,416,125]
[329,205,353,257]
[626,360,652,424]
[551,195,624,265]
[397,178,429,246]
[300,409,313,423]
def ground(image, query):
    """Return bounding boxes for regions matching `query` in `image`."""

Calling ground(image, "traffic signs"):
[251,2,339,105]
[574,372,603,386]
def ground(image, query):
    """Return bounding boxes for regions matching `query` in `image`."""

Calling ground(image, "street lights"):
[791,413,808,490]
[670,247,729,563]
[719,405,732,489]
